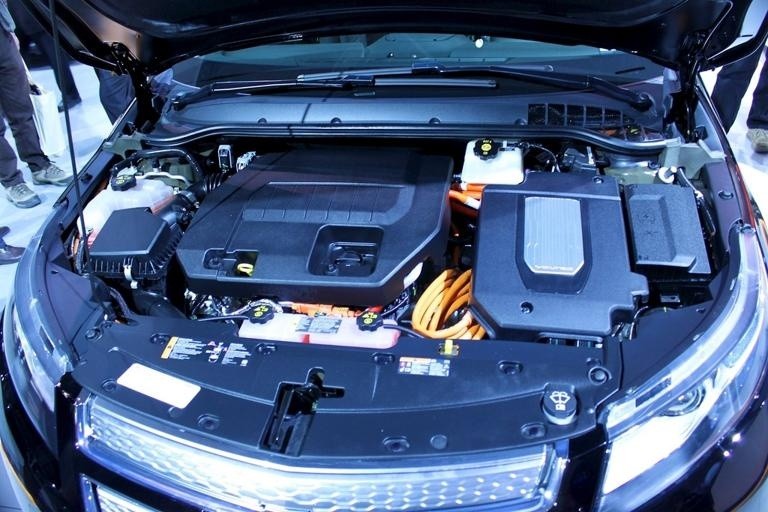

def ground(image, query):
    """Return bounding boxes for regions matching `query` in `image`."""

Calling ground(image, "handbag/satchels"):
[18,51,68,162]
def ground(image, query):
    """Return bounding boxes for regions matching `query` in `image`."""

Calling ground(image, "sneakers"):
[31,162,75,187]
[57,92,82,114]
[746,128,768,153]
[4,180,42,209]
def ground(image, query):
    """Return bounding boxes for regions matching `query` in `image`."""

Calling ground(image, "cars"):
[0,0,768,512]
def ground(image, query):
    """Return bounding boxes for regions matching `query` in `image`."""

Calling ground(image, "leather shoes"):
[0,244,26,265]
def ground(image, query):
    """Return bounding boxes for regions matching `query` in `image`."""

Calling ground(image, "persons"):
[0,0,79,210]
[8,0,84,113]
[707,44,767,159]
[0,225,27,268]
